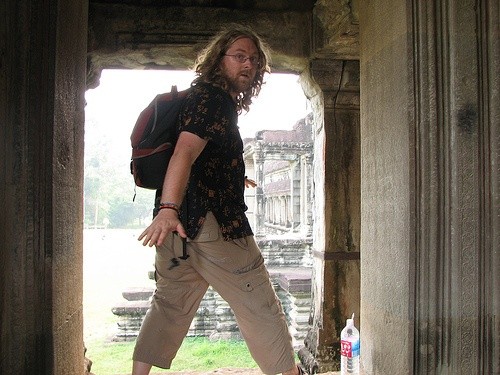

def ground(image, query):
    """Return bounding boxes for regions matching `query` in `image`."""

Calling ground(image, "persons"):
[125,26,311,375]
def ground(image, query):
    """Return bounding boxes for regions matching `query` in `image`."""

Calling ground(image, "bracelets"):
[156,200,181,208]
[155,206,179,214]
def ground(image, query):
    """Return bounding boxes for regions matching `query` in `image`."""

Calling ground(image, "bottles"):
[340,312,360,375]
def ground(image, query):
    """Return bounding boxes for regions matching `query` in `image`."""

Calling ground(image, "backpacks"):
[127,84,195,190]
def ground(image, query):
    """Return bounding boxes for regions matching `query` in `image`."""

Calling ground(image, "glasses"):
[224,54,261,64]
